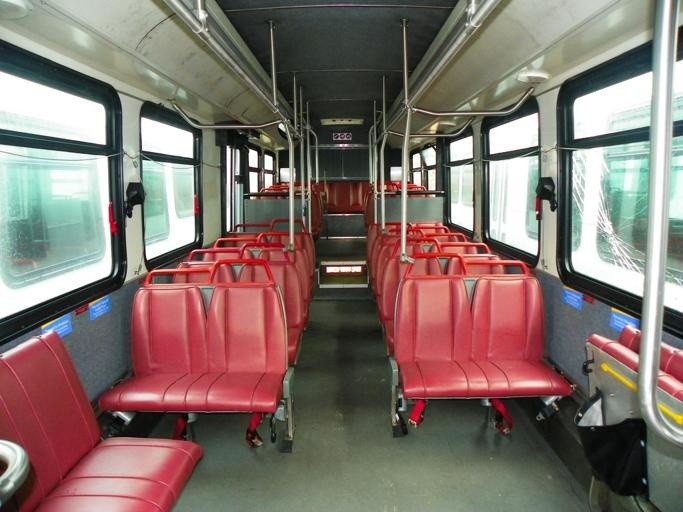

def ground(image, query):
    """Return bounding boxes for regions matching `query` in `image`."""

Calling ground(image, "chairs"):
[366,222,574,437]
[98,220,314,453]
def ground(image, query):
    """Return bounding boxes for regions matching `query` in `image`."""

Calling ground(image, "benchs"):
[0,329,206,512]
[258,179,431,240]
